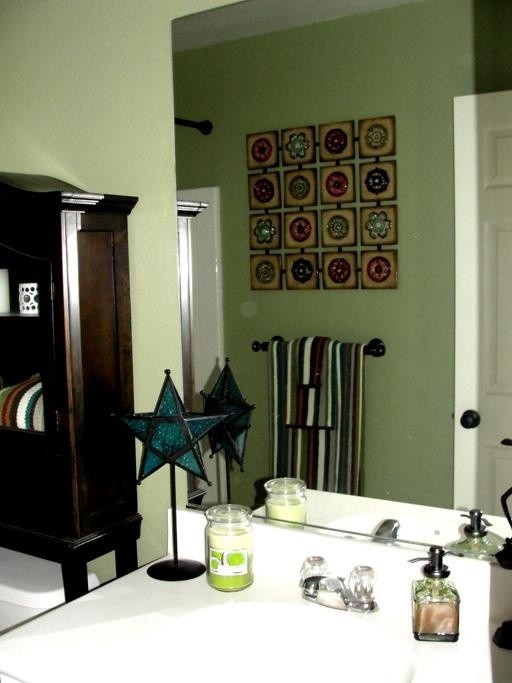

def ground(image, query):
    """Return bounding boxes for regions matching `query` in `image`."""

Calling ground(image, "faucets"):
[372,519,400,547]
[302,575,352,609]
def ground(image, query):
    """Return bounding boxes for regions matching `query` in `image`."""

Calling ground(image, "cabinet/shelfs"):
[0,171,139,607]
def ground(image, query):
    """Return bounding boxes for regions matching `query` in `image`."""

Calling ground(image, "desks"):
[249,481,512,563]
[0,538,511,678]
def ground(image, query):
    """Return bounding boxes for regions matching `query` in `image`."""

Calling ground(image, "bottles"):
[411,544,460,642]
[204,503,255,594]
[264,477,308,530]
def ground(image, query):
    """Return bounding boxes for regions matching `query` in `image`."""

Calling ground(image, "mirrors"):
[173,3,512,527]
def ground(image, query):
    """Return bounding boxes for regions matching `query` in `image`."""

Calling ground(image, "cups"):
[18,282,40,315]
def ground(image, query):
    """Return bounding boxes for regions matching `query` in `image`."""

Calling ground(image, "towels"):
[267,331,366,499]
